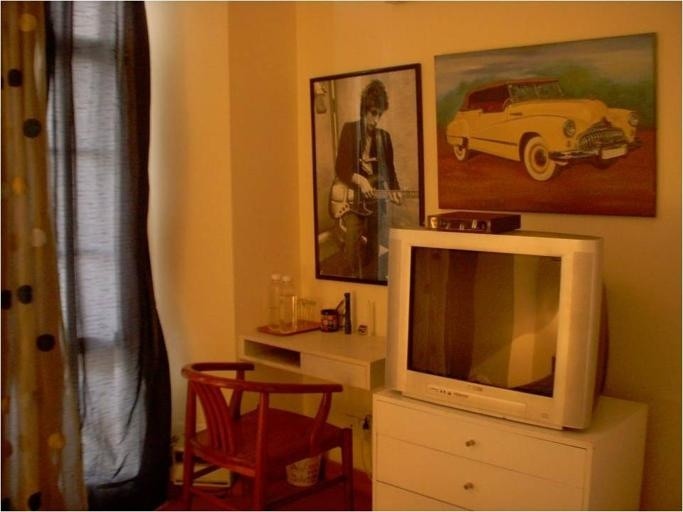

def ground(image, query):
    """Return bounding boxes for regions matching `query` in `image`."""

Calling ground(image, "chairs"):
[177,361,354,512]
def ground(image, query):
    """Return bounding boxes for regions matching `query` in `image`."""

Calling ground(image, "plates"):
[256,319,320,337]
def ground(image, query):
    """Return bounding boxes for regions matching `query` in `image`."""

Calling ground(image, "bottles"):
[277,274,298,333]
[321,308,340,333]
[266,273,282,330]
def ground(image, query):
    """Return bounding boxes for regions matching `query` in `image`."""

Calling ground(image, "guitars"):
[329,175,419,219]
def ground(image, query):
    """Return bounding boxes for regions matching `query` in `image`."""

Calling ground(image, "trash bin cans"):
[286,456,322,487]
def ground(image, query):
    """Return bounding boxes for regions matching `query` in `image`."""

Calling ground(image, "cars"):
[445,78,640,181]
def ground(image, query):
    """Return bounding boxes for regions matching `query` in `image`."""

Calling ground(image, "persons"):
[334,79,404,280]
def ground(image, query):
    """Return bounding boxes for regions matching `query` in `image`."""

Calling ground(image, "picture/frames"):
[309,62,425,285]
[434,31,658,219]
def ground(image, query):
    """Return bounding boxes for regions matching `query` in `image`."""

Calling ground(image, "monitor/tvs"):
[385,227,609,432]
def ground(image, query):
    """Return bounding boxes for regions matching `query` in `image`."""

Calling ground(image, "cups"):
[303,302,315,329]
[298,299,306,327]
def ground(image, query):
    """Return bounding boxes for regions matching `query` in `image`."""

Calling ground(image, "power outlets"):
[359,417,370,438]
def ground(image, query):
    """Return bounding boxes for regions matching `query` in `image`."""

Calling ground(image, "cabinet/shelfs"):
[370,387,648,511]
[238,319,386,477]
